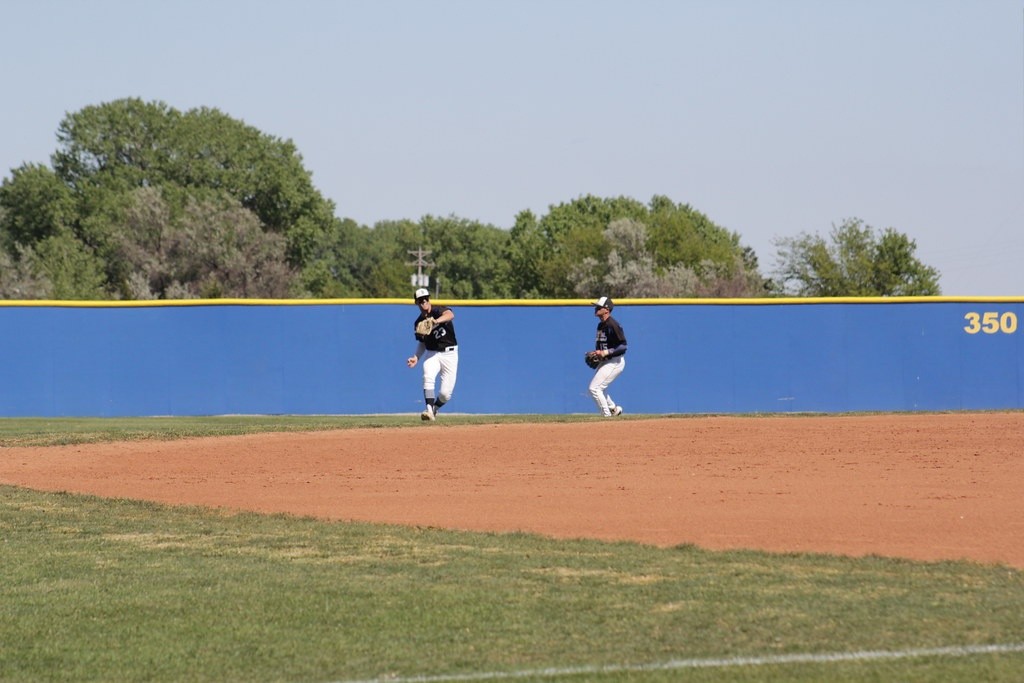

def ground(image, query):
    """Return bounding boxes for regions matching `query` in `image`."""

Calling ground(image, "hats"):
[414,288,431,300]
[591,296,615,312]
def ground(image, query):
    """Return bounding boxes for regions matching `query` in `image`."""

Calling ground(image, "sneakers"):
[422,409,437,421]
[611,406,622,415]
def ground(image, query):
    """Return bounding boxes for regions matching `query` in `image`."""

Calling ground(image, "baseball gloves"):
[584,349,605,370]
[415,316,439,336]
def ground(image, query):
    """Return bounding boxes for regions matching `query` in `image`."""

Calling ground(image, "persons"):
[407,288,458,421]
[584,297,628,417]
